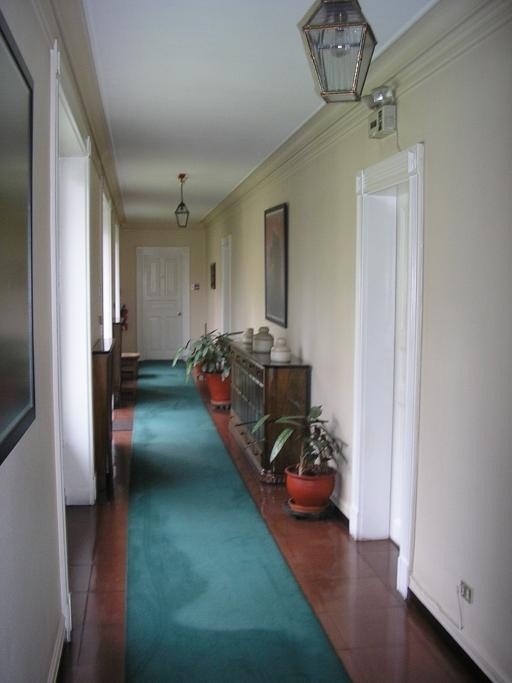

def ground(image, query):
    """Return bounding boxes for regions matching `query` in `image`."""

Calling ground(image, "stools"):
[121,348,141,380]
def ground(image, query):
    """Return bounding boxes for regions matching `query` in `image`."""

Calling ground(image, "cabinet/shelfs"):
[227,339,313,482]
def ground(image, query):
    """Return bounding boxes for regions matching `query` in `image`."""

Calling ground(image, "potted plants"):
[171,322,245,410]
[233,398,340,519]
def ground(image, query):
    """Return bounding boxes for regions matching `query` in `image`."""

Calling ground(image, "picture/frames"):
[260,198,291,329]
[0,11,37,473]
[209,261,217,290]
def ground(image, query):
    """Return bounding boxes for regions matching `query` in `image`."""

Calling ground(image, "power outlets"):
[457,577,478,606]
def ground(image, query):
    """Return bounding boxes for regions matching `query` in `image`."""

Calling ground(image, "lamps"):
[174,169,193,229]
[295,0,380,104]
